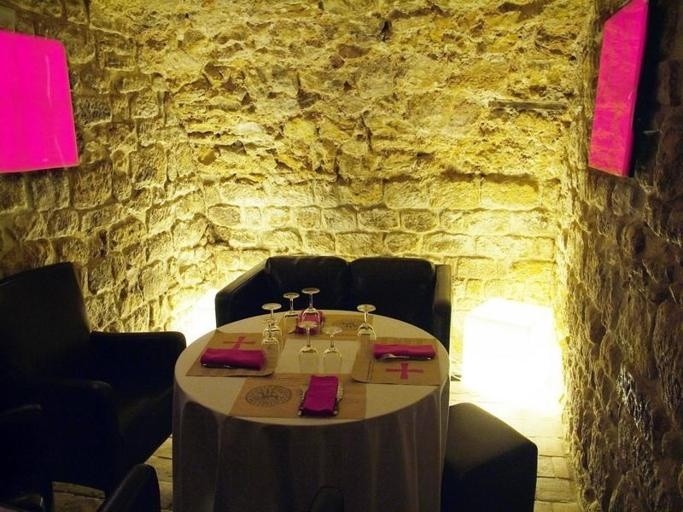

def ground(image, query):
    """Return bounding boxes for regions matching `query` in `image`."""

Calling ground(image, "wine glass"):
[355,304,376,351]
[300,286,321,321]
[259,302,284,362]
[282,293,298,336]
[295,320,319,377]
[320,326,344,378]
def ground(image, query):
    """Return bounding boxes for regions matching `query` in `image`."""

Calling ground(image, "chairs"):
[0,260,185,464]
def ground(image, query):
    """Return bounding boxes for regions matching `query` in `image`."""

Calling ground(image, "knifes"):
[333,381,344,415]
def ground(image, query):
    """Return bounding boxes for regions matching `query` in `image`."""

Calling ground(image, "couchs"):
[437,401,539,511]
[214,254,452,352]
[0,404,161,512]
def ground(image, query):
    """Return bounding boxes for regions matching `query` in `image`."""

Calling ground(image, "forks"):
[378,353,431,361]
[296,381,309,416]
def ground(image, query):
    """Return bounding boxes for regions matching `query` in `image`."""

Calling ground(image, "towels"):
[298,374,339,418]
[373,344,434,359]
[294,309,325,337]
[199,348,267,371]
